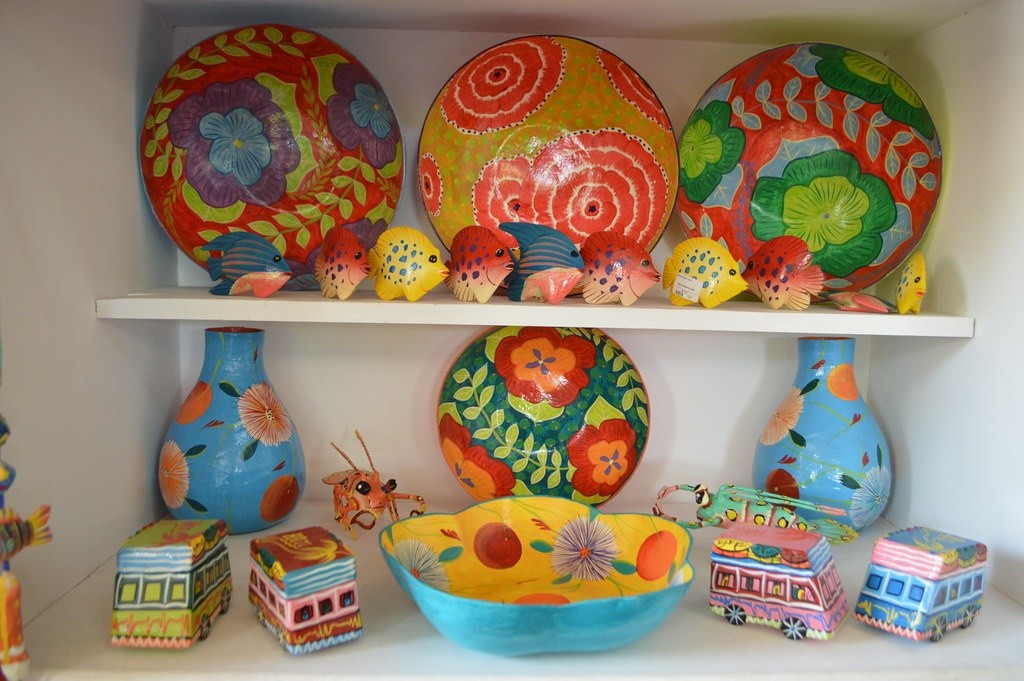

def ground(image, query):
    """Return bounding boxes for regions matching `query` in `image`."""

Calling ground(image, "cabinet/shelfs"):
[0,1,1024,681]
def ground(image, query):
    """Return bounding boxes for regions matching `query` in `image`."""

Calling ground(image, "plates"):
[139,23,406,291]
[378,493,696,657]
[436,326,650,511]
[416,34,680,299]
[674,41,942,303]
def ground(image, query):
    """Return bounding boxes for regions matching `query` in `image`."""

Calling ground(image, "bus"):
[852,525,988,642]
[106,519,236,651]
[247,526,365,656]
[706,521,851,643]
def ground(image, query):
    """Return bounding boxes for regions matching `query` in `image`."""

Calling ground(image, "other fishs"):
[199,220,928,317]
